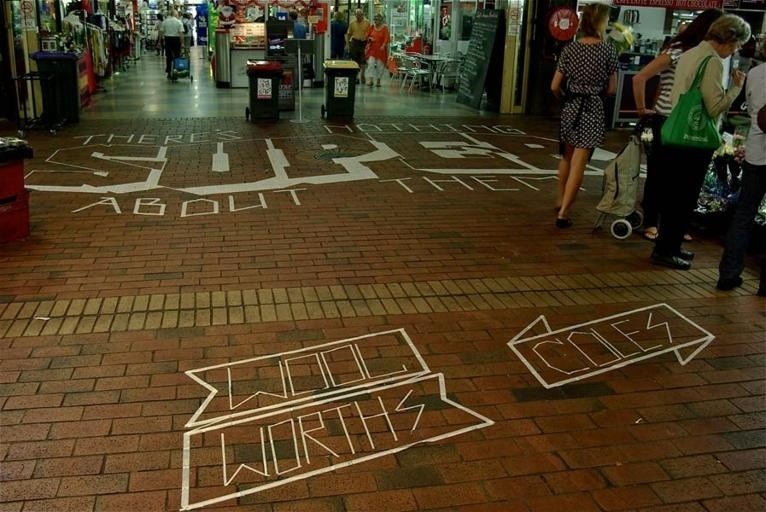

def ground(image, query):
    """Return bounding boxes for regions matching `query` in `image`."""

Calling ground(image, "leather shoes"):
[651,247,695,270]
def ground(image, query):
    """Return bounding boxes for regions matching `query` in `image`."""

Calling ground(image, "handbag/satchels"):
[660,90,722,148]
[364,36,375,54]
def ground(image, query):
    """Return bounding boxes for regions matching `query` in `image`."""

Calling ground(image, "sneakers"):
[556,205,573,227]
[717,276,742,291]
[356,78,381,87]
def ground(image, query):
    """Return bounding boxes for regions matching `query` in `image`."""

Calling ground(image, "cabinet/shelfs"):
[611,72,662,129]
[139,12,162,39]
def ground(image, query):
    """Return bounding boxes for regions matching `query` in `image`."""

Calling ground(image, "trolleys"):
[592,112,654,240]
[169,47,194,82]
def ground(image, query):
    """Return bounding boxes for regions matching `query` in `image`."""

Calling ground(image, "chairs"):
[392,49,463,97]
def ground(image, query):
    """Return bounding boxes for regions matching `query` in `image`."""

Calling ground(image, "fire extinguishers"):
[424,43,432,55]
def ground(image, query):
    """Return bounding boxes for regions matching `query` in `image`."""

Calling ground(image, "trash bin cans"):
[321,59,360,122]
[0,136,33,244]
[29,50,80,124]
[246,58,283,124]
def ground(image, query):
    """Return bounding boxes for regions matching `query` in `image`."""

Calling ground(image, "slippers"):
[642,228,659,241]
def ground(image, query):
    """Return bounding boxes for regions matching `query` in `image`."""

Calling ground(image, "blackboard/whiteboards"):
[455,9,499,110]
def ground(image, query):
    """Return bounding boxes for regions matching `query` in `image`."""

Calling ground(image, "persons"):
[347,8,371,84]
[177,11,196,52]
[649,15,755,270]
[288,11,306,39]
[367,14,392,87]
[161,10,185,74]
[630,9,725,244]
[549,3,621,228]
[153,14,165,56]
[717,61,766,296]
[330,11,348,60]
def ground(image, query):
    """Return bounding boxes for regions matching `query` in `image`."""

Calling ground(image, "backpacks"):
[182,20,189,34]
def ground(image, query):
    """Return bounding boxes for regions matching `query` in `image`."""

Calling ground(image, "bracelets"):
[636,106,647,111]
